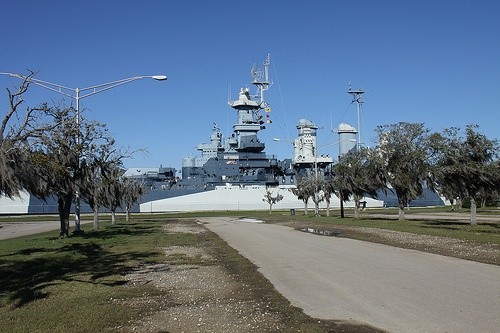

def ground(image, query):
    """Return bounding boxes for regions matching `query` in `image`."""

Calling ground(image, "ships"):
[0,52,459,216]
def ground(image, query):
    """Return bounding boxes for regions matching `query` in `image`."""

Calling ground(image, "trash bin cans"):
[290,208,296,215]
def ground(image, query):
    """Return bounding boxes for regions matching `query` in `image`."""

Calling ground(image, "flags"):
[265,107,271,112]
[265,103,270,107]
[267,119,272,124]
[266,113,271,119]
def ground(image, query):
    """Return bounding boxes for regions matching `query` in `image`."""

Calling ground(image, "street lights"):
[1,69,168,235]
